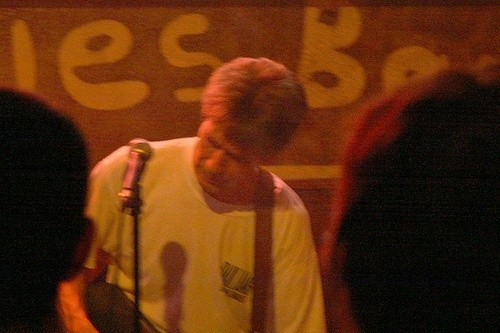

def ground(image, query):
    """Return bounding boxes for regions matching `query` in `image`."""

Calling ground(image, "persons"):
[0,86,99,332]
[55,56,328,332]
[322,68,499,332]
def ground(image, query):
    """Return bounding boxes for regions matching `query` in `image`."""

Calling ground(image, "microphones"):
[117,137,153,219]
